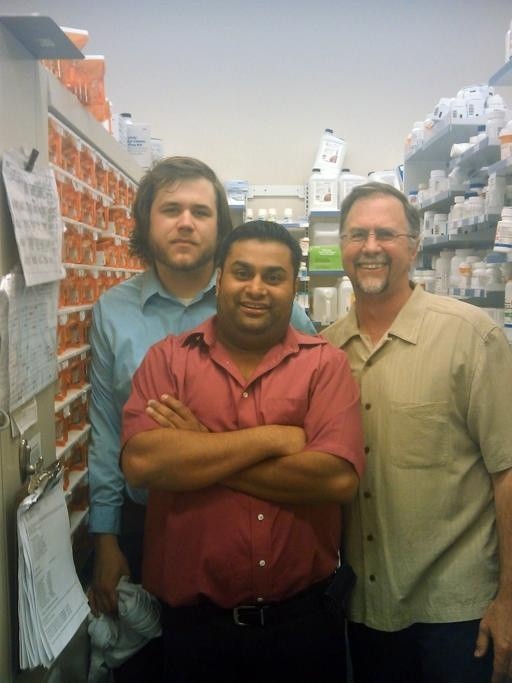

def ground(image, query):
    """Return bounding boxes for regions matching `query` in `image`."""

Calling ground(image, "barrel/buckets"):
[311,128,401,324]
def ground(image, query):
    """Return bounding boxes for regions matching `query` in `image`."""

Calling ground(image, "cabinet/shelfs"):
[38,58,166,683]
[221,200,356,330]
[398,55,512,353]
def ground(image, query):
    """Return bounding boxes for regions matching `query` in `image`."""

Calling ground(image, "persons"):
[117,220,367,681]
[317,176,511,680]
[81,153,320,683]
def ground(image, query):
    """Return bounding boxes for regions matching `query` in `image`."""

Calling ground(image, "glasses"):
[343,230,416,246]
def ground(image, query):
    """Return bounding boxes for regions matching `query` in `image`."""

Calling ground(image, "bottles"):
[404,84,512,330]
[505,19,512,60]
[246,207,310,281]
[122,113,163,176]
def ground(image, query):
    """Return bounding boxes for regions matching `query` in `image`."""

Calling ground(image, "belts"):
[226,563,358,628]
